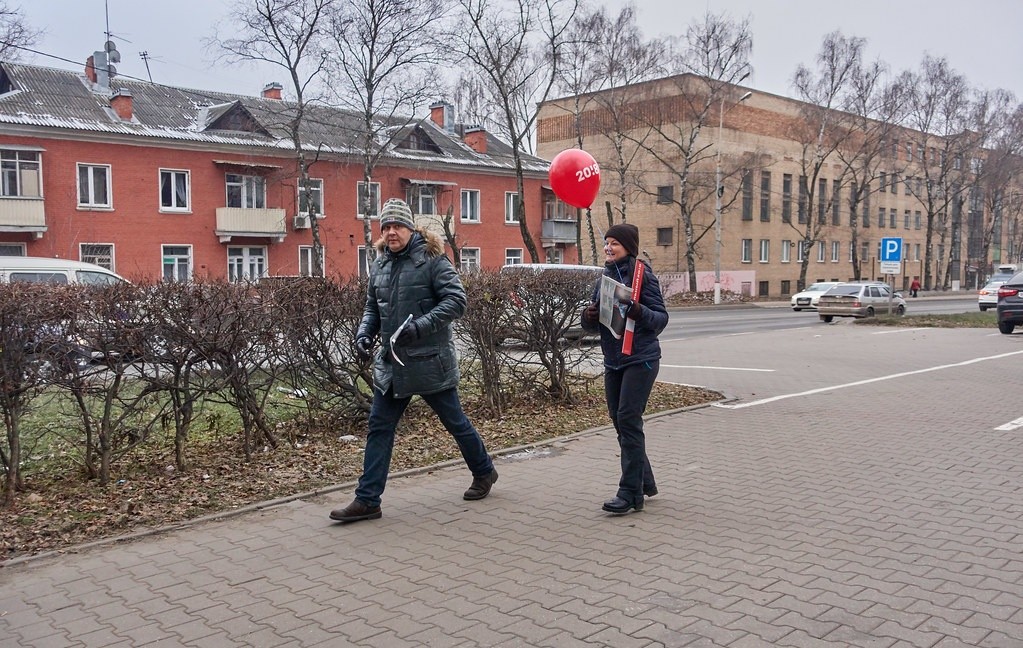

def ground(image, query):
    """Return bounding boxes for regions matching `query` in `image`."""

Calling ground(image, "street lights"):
[713,91,753,303]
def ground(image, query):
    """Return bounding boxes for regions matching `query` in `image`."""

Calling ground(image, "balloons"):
[549,148,600,209]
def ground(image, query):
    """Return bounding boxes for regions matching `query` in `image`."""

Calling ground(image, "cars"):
[817,284,906,323]
[490,265,605,347]
[0,255,174,372]
[978,281,1008,312]
[790,282,847,312]
[996,270,1023,335]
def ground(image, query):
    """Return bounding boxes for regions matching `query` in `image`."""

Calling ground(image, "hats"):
[604,224,638,258]
[380,198,414,233]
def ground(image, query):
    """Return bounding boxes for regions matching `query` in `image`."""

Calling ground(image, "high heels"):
[643,482,658,497]
[603,495,644,513]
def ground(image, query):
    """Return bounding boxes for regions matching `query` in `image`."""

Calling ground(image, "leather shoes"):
[463,468,500,500]
[330,499,382,521]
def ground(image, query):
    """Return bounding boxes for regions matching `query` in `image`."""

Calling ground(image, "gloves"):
[583,299,600,324]
[394,321,418,347]
[618,298,643,322]
[356,336,372,361]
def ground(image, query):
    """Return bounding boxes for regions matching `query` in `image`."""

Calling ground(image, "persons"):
[581,224,669,513]
[911,278,920,297]
[329,198,499,520]
